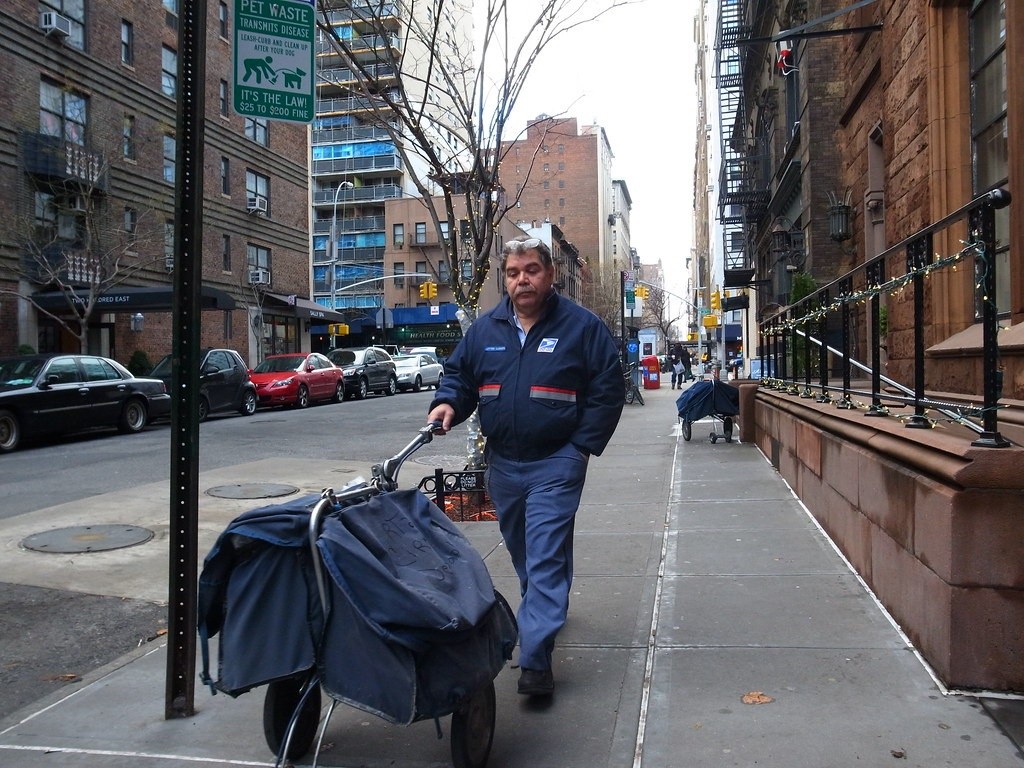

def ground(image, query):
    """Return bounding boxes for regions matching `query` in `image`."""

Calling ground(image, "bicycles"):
[622,361,645,406]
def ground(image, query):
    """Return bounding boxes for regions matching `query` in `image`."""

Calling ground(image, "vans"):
[372,344,438,365]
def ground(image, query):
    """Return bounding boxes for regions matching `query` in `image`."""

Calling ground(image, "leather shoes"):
[516,666,554,692]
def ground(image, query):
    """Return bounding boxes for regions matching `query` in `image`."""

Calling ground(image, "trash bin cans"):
[642,356,660,390]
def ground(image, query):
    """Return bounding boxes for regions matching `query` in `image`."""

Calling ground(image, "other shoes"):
[692,378,695,381]
[677,386,682,389]
[672,384,675,389]
[682,381,686,383]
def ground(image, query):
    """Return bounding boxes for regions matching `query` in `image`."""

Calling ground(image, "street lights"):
[329,182,354,348]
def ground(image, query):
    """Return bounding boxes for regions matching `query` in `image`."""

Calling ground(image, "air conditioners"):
[706,185,714,193]
[706,134,710,141]
[704,124,712,131]
[247,198,268,212]
[774,29,793,61]
[701,153,706,160]
[249,271,271,284]
[40,11,71,37]
[699,112,704,118]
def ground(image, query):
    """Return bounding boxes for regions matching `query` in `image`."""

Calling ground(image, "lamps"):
[772,216,805,270]
[129,313,144,333]
[824,187,857,257]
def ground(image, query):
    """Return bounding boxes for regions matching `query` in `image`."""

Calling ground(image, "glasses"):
[504,238,547,249]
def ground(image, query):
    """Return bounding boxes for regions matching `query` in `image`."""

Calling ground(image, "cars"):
[148,345,260,424]
[0,352,173,455]
[656,353,676,374]
[390,353,444,393]
[246,352,346,411]
[704,353,775,380]
[320,346,398,401]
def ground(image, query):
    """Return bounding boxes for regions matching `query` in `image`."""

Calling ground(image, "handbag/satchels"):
[673,357,685,374]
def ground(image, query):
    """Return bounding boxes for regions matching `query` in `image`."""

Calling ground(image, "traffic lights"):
[688,333,696,341]
[634,287,639,297]
[642,286,650,300]
[711,291,721,310]
[419,281,429,299]
[429,282,438,299]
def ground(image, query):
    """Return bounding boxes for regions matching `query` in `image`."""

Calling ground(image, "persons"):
[667,343,683,389]
[659,358,668,374]
[428,235,625,696]
[682,346,695,383]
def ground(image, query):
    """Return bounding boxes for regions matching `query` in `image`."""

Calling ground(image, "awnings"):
[260,292,345,326]
[32,286,236,312]
